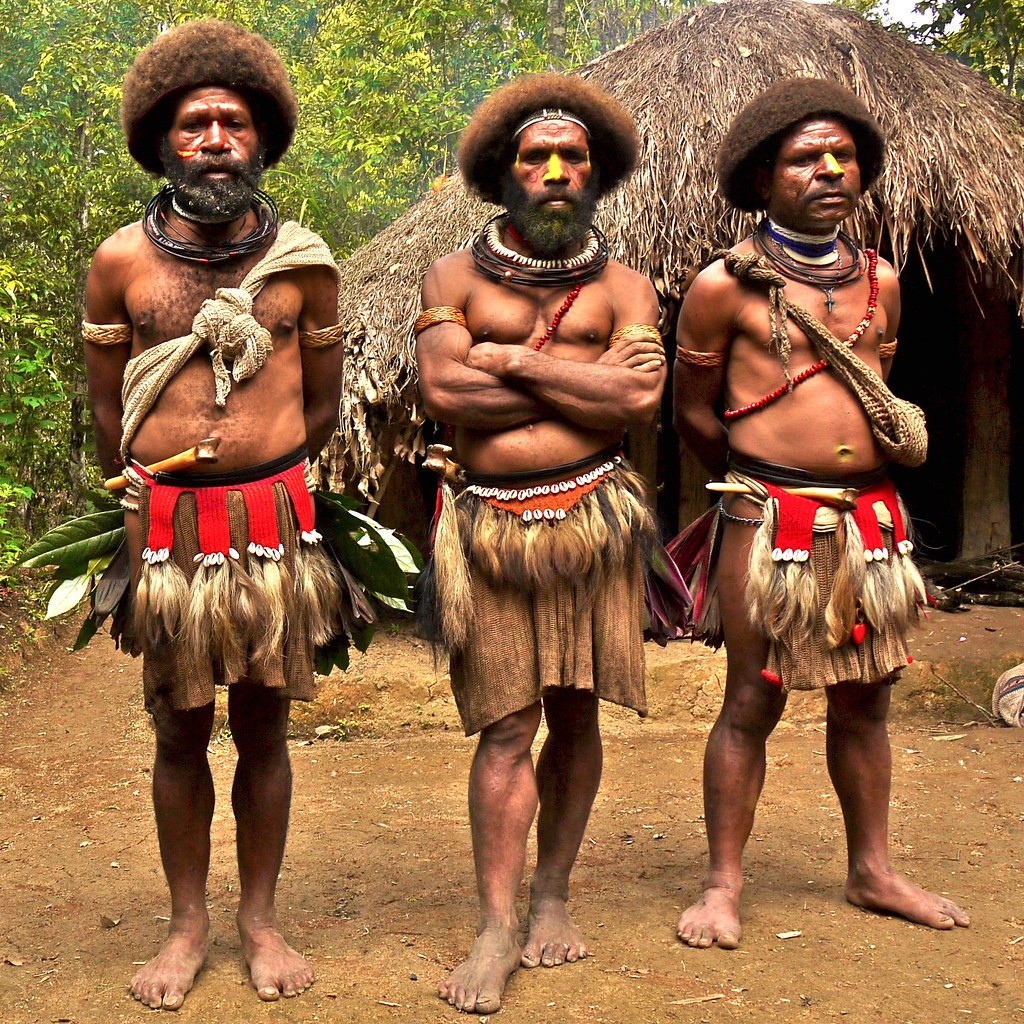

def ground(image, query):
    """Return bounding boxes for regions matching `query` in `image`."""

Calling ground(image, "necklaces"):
[469,213,612,292]
[753,218,868,314]
[142,185,280,268]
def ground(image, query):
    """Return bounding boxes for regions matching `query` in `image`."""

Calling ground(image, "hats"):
[718,78,889,212]
[119,21,298,173]
[457,74,637,205]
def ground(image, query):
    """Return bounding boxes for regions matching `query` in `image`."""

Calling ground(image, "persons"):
[410,72,668,1017]
[71,17,344,1013]
[667,73,973,949]
